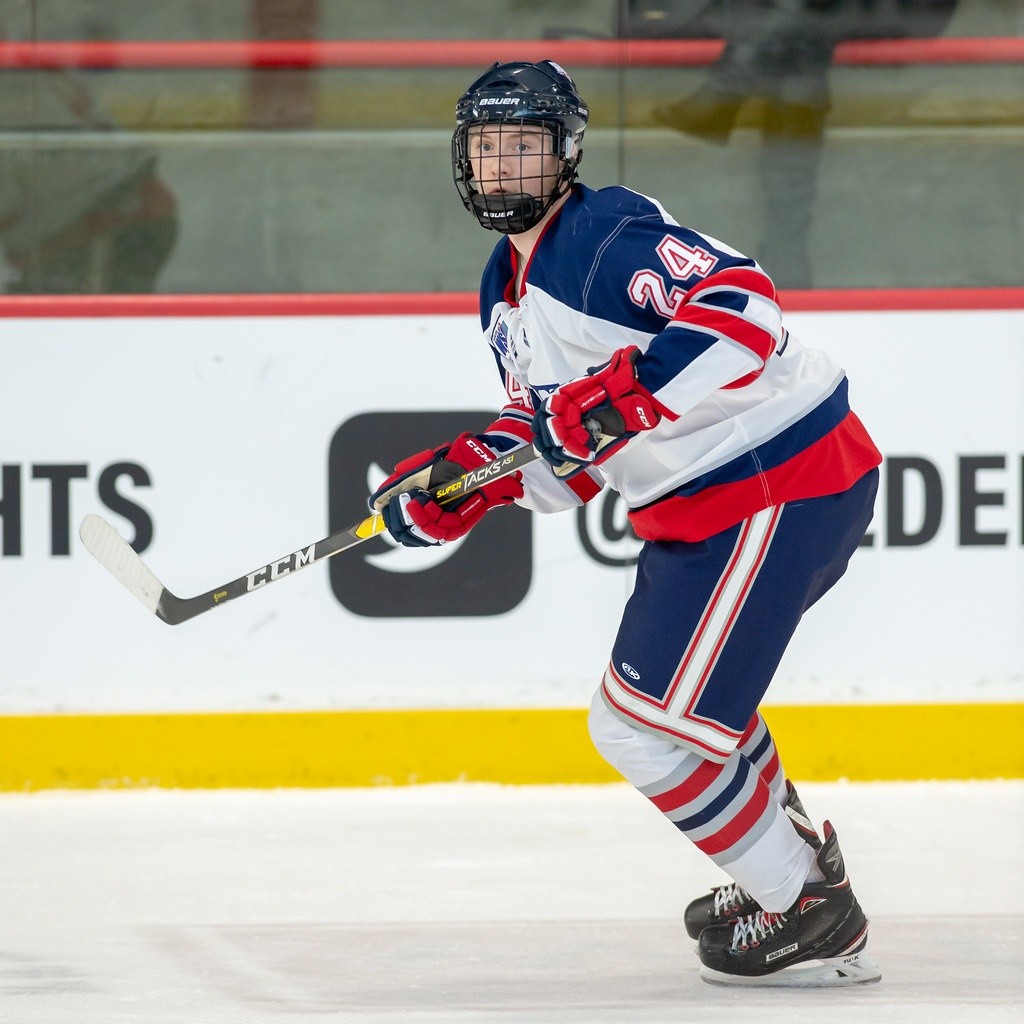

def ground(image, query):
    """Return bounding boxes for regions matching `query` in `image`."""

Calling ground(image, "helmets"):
[451,60,591,235]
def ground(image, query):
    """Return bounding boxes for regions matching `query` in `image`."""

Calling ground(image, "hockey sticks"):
[72,406,606,636]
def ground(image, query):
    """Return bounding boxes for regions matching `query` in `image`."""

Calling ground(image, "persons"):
[623,1,959,291]
[368,60,882,987]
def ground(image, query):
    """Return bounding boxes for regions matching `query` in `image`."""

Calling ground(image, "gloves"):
[533,344,657,481]
[370,431,526,549]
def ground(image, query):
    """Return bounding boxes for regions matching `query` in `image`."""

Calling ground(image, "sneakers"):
[681,776,883,989]
